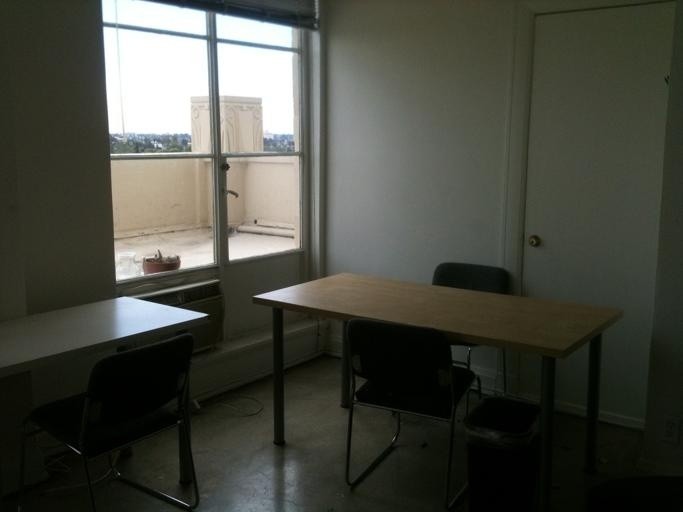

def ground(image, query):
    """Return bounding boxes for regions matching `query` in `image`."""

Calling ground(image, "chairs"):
[344,318,482,510]
[428,262,514,419]
[17,334,201,512]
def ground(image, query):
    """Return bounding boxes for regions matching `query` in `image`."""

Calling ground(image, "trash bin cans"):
[464,394,540,512]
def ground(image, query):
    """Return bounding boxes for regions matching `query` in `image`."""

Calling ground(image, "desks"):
[254,275,624,512]
[0,295,212,512]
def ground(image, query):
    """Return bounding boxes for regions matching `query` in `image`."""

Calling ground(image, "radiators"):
[119,278,226,352]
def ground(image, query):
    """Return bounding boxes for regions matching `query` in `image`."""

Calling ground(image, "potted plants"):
[143,250,181,276]
